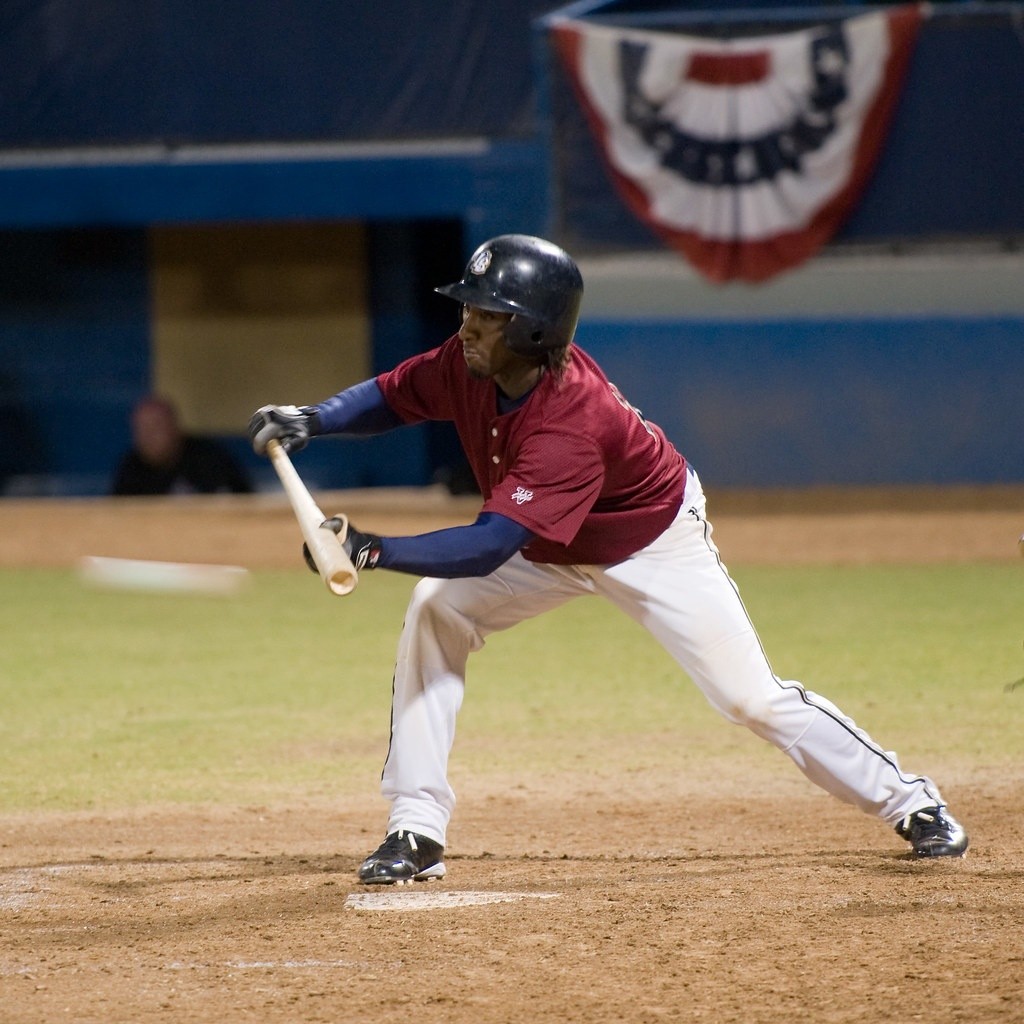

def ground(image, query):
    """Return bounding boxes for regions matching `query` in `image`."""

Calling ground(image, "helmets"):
[435,234,585,348]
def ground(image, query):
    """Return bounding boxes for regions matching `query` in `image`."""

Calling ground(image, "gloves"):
[249,404,321,456]
[303,512,384,575]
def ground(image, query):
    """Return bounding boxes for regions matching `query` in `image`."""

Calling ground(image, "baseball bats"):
[265,437,360,597]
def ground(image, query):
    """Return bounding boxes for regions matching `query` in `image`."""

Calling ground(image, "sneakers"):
[358,828,446,883]
[896,805,970,859]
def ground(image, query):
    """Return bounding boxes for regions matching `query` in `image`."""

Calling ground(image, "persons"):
[249,232,969,884]
[113,399,257,494]
